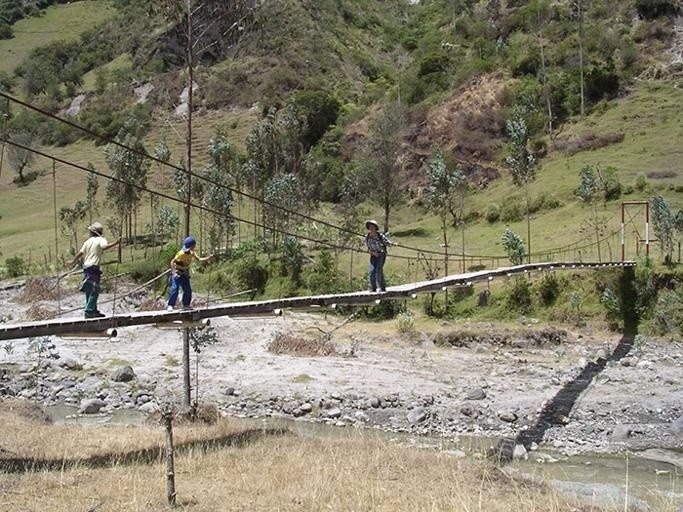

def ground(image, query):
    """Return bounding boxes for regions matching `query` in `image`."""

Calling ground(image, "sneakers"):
[167,305,174,311]
[84,308,104,318]
[183,305,192,310]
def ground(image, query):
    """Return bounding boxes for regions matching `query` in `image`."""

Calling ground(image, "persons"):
[65,221,121,319]
[366,219,398,293]
[166,236,213,312]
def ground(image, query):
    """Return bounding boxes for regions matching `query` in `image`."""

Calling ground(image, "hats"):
[88,222,103,236]
[366,220,379,231]
[183,238,196,251]
